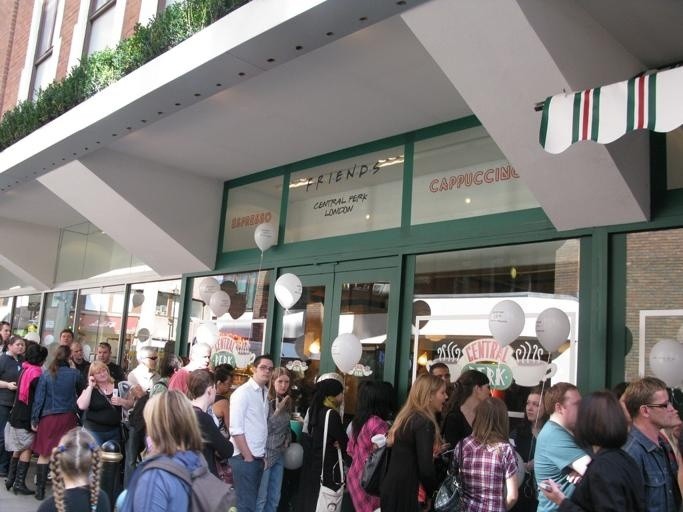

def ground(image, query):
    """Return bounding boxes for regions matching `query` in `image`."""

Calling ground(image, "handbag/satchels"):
[314,485,346,511]
[9,399,33,429]
[433,441,469,511]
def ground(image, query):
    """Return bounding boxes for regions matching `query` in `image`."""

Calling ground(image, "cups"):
[370,433,387,449]
[113,388,119,397]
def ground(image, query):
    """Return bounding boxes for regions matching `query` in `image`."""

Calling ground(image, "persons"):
[1,319,683,512]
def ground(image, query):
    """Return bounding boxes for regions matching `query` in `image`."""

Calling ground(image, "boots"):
[34,463,49,501]
[5,457,36,495]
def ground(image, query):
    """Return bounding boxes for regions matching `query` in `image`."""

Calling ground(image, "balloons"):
[228,294,246,320]
[133,292,145,308]
[488,299,525,348]
[199,277,220,304]
[209,290,231,318]
[254,223,276,252]
[274,273,303,311]
[220,280,237,296]
[534,307,571,353]
[412,300,431,330]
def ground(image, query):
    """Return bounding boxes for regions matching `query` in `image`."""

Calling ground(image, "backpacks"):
[361,429,393,499]
[139,450,237,512]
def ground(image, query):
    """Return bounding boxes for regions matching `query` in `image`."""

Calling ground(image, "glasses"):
[641,402,673,410]
